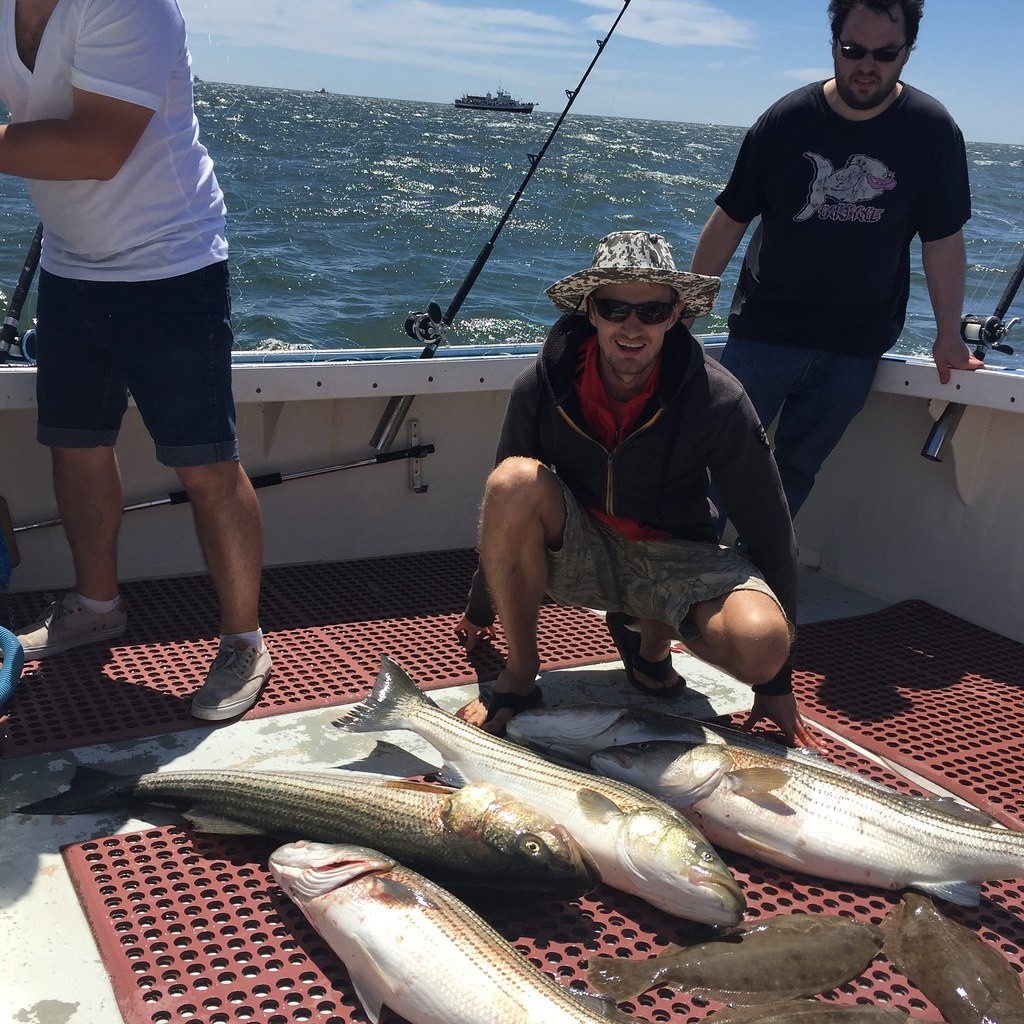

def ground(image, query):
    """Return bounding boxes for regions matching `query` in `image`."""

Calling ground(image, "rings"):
[803,724,807,729]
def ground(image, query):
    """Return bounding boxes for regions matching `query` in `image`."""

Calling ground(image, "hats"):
[542,231,721,319]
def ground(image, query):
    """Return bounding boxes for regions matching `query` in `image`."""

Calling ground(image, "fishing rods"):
[368,1,628,449]
[1,220,48,364]
[922,253,1021,461]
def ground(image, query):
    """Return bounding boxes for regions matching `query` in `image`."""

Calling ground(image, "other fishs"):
[12,648,1024,1024]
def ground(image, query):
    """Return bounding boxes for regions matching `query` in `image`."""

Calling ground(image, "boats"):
[453,86,539,112]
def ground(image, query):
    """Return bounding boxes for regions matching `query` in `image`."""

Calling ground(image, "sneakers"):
[189,639,273,721]
[0,593,128,662]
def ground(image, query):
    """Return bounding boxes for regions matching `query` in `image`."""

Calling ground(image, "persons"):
[1,0,274,721]
[454,233,831,756]
[678,0,985,577]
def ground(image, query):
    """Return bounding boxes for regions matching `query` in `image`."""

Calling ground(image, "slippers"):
[453,683,543,738]
[606,610,686,698]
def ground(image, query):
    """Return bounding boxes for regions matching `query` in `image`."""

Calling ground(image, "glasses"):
[589,293,680,325]
[838,35,908,63]
